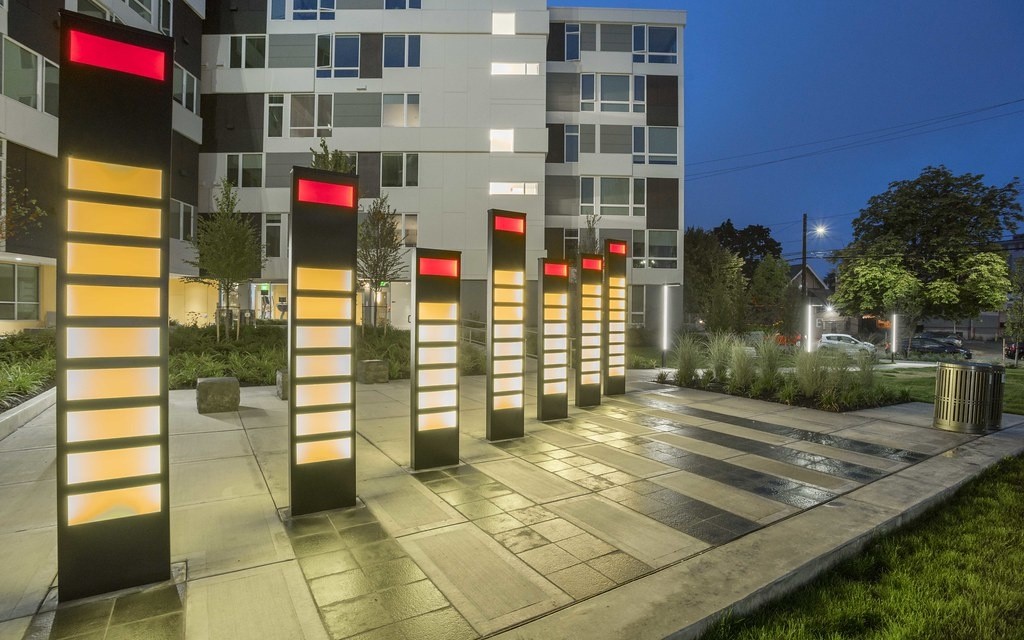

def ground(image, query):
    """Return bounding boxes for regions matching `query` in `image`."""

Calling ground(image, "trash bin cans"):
[934,361,1006,432]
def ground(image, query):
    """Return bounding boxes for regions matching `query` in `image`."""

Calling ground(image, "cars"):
[884,337,972,359]
[913,331,964,349]
[817,333,878,356]
[1004,341,1024,361]
